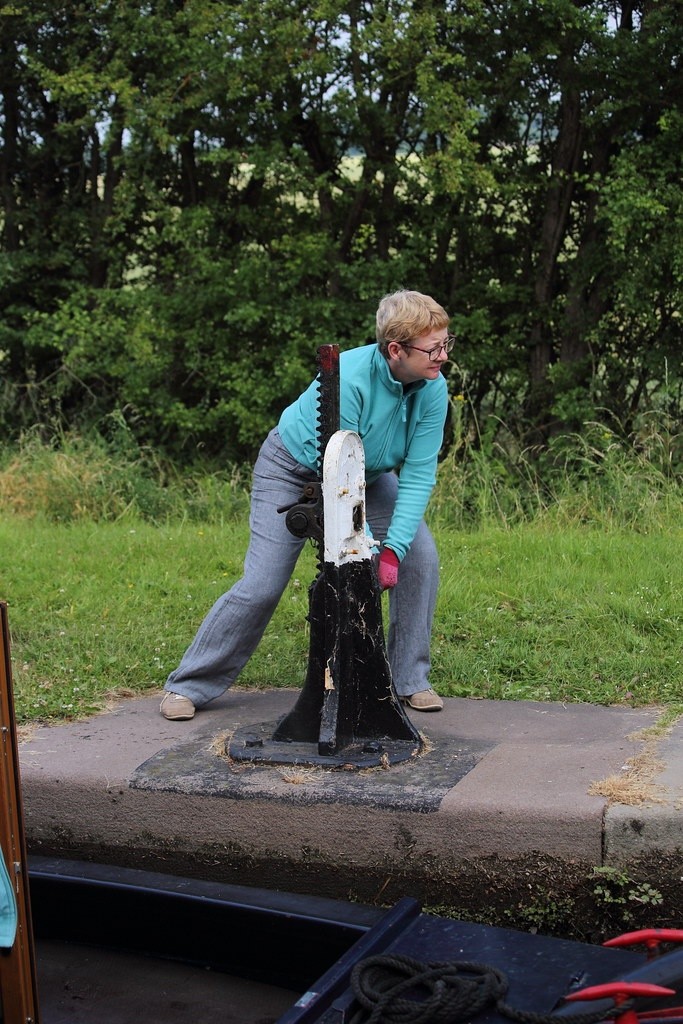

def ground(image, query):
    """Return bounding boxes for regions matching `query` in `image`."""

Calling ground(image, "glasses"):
[399,337,455,362]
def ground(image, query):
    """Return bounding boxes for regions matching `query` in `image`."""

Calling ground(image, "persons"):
[157,290,449,721]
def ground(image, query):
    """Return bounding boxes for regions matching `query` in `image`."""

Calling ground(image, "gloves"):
[376,548,398,591]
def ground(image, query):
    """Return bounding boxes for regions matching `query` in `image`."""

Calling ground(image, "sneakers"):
[400,688,443,711]
[159,692,195,719]
